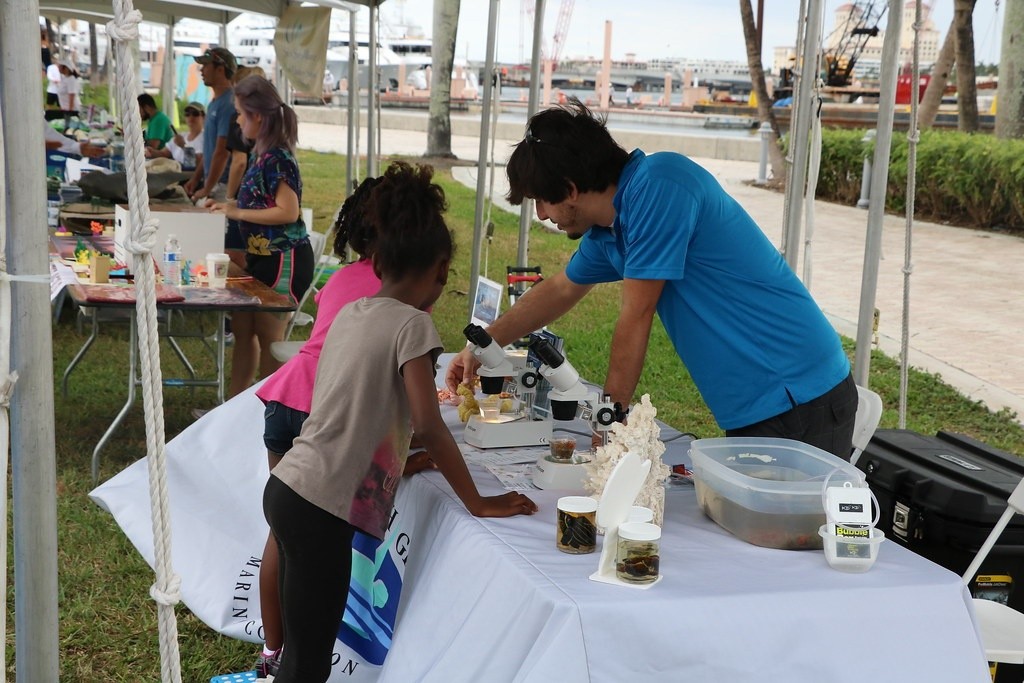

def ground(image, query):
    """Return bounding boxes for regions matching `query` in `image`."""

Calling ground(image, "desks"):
[88,350,996,683]
[48,225,299,494]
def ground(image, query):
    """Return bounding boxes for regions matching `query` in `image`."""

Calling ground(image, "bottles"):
[110,132,124,171]
[163,234,182,288]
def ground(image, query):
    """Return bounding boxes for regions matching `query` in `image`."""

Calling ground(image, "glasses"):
[525,115,580,154]
[204,48,226,62]
[184,110,201,117]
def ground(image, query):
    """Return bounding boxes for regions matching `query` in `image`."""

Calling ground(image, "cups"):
[478,398,502,419]
[557,496,597,553]
[549,435,576,460]
[616,522,661,584]
[205,254,230,291]
[629,505,654,524]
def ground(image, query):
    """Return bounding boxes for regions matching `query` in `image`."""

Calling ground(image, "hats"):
[56,57,74,73]
[183,101,206,115]
[192,47,237,73]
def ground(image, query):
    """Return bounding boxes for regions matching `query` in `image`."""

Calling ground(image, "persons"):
[189,76,314,421]
[45,120,106,162]
[182,48,272,342]
[248,160,541,682]
[43,51,85,120]
[444,107,860,463]
[260,207,540,683]
[136,93,177,151]
[144,102,208,193]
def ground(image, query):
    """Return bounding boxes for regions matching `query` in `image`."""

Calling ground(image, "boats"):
[76,23,432,88]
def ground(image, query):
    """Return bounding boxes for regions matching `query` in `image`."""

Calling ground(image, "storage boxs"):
[686,438,866,551]
[89,254,110,284]
[112,203,227,278]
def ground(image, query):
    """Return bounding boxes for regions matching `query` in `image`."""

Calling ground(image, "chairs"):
[267,228,336,365]
[848,386,884,466]
[964,470,1024,666]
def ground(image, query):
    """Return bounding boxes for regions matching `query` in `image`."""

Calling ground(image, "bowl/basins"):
[818,524,886,573]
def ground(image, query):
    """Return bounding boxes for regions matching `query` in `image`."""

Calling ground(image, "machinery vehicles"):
[778,0,888,103]
